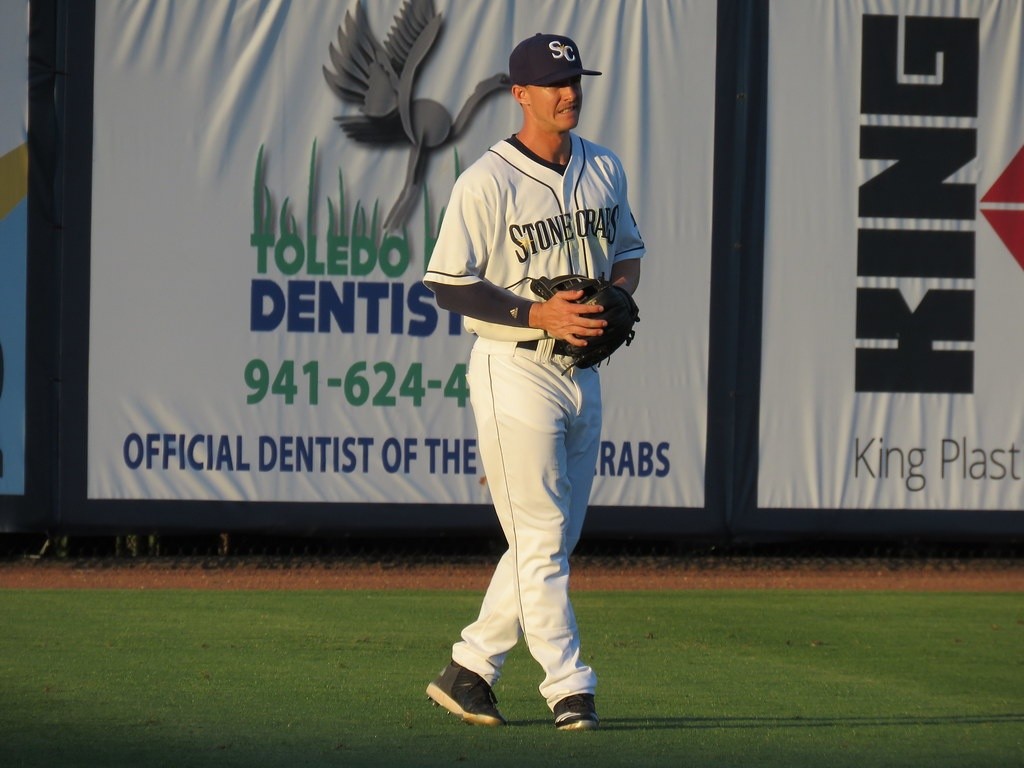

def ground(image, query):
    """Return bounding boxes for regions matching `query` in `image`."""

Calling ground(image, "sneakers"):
[554,693,600,731]
[426,661,506,726]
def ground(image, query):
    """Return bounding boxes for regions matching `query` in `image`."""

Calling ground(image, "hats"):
[509,33,602,85]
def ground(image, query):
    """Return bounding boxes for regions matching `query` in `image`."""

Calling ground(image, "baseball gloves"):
[530,273,640,370]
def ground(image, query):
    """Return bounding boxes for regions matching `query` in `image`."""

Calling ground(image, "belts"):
[515,339,572,357]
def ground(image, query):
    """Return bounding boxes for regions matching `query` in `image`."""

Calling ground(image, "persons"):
[425,32,646,731]
